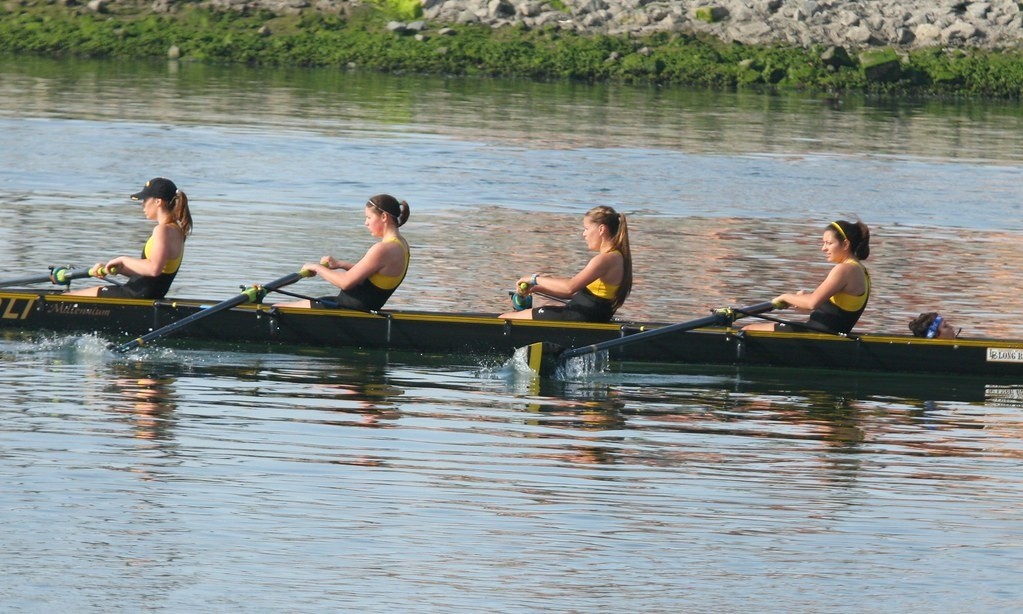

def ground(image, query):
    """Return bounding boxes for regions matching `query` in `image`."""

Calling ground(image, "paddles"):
[506,281,532,310]
[0,266,118,286]
[104,262,329,354]
[512,300,790,377]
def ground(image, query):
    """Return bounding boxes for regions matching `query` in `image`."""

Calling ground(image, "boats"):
[0,284,1023,377]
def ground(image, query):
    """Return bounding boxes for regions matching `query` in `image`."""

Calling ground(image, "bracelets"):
[531,273,539,285]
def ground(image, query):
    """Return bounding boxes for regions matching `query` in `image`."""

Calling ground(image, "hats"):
[130,178,178,201]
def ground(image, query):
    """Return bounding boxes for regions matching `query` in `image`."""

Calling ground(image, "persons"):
[739,217,873,333]
[497,207,634,323]
[270,193,411,312]
[60,176,193,298]
[906,312,955,341]
